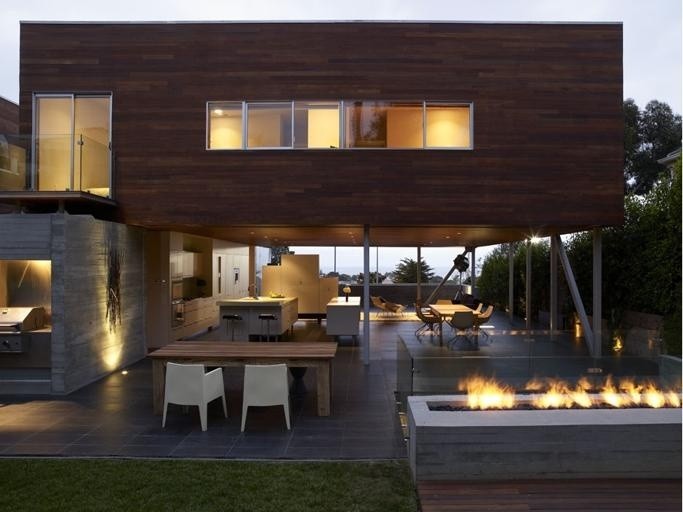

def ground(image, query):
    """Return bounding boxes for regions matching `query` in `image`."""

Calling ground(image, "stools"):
[258,313,279,342]
[222,314,242,342]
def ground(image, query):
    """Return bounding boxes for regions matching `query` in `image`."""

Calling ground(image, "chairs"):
[240,363,292,433]
[371,296,406,320]
[413,300,494,351]
[161,362,228,431]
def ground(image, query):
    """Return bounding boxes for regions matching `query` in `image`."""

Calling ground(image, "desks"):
[145,340,339,417]
